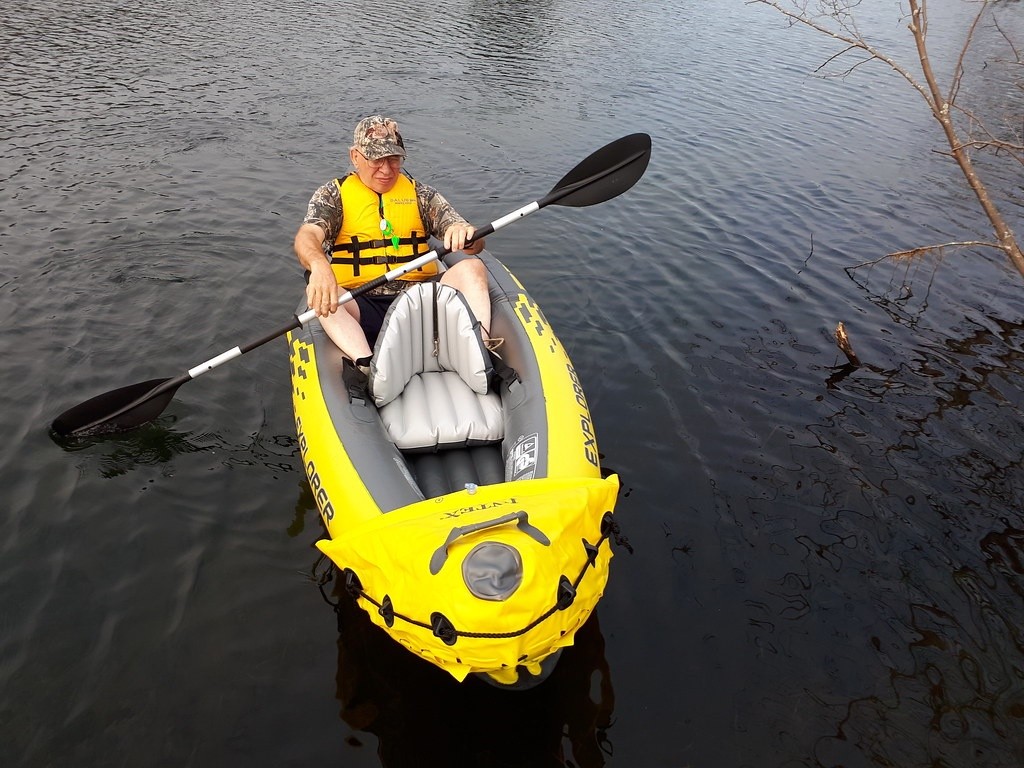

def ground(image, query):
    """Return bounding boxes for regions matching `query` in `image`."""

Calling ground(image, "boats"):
[286,241,625,692]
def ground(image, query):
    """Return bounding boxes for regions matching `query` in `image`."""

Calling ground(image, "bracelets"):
[463,242,475,250]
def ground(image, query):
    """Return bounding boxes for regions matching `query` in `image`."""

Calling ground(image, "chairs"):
[367,282,506,451]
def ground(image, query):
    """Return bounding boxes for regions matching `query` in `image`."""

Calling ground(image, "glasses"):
[355,149,405,169]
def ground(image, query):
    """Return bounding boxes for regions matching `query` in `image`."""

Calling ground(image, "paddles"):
[49,130,655,434]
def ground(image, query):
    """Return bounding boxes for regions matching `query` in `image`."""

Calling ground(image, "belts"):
[363,282,420,296]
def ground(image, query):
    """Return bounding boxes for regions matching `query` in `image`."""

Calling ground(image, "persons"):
[293,114,503,386]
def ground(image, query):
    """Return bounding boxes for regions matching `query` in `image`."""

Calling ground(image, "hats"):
[353,115,406,160]
